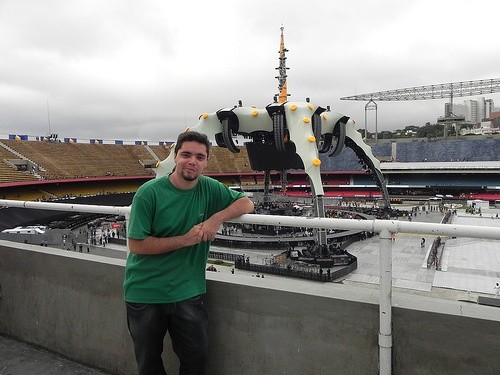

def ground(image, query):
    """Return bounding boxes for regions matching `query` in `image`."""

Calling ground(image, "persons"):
[123,131,254,375]
[24,157,500,299]
